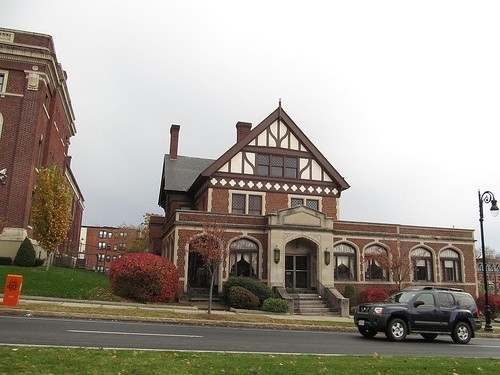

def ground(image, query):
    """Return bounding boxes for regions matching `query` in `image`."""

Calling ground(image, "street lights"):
[477,189,500,334]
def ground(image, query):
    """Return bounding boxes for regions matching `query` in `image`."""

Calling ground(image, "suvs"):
[354,285,478,344]
